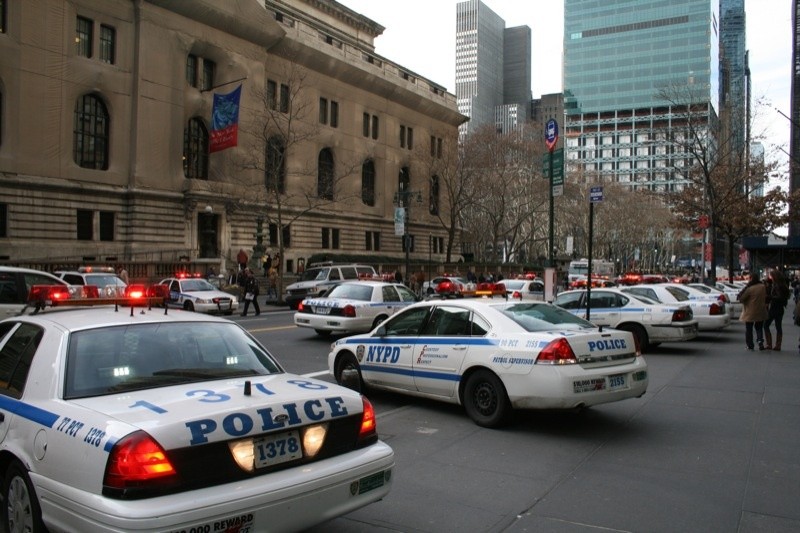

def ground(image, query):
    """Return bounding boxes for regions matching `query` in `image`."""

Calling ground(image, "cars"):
[422,272,477,297]
[327,281,649,428]
[604,273,751,331]
[0,266,78,320]
[51,265,144,307]
[152,272,240,316]
[292,273,423,336]
[554,278,700,353]
[490,273,545,301]
[0,283,395,533]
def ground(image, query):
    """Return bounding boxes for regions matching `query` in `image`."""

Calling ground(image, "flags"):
[208,82,242,155]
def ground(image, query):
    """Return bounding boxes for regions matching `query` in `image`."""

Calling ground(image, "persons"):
[761,269,790,351]
[409,272,426,292]
[228,249,279,317]
[466,269,506,283]
[395,268,403,283]
[736,268,770,352]
[119,267,129,285]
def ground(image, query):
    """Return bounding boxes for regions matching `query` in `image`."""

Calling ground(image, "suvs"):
[282,261,378,309]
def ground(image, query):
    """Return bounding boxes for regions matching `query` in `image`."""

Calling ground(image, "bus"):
[567,258,615,289]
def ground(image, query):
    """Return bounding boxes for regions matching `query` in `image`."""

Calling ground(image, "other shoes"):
[766,346,772,349]
[774,347,781,351]
[758,340,764,350]
